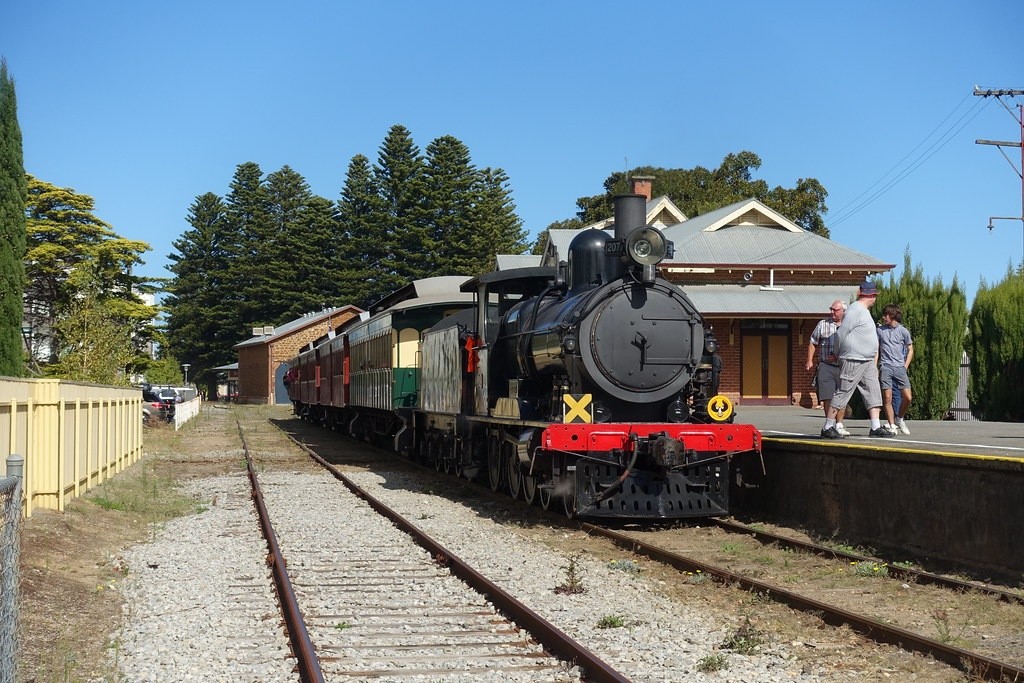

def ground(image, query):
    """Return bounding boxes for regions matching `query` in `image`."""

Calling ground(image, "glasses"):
[829,307,840,312]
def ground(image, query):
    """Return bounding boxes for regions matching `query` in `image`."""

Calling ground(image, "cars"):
[142,387,194,424]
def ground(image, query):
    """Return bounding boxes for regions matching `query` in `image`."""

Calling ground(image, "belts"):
[846,359,872,364]
[820,361,839,367]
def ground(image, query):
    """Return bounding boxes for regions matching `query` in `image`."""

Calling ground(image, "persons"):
[283,370,290,395]
[874,304,914,436]
[805,281,895,440]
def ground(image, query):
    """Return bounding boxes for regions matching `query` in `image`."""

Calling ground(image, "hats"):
[857,281,880,297]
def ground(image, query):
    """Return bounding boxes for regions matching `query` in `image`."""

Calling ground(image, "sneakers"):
[895,418,910,434]
[881,423,899,429]
[869,426,896,438]
[821,426,845,439]
[834,424,850,436]
[889,425,897,435]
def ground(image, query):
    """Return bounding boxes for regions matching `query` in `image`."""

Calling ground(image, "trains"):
[282,192,764,523]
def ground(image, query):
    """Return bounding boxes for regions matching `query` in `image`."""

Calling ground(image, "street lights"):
[180,363,192,383]
